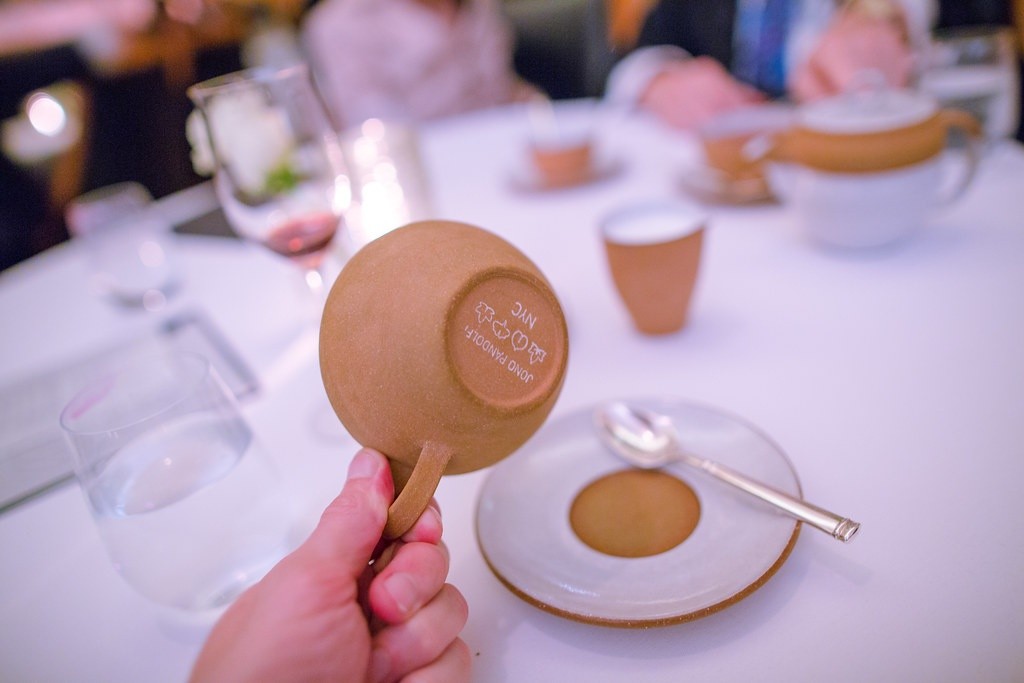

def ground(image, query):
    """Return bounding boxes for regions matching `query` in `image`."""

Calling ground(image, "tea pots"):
[707,68,980,246]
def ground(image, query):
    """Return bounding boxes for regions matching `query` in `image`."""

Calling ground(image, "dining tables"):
[2,100,1024,683]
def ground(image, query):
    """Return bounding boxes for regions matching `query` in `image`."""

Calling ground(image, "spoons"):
[590,390,861,541]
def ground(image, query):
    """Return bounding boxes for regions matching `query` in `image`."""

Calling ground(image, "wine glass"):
[188,64,353,300]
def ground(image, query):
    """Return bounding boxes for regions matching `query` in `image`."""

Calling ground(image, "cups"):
[63,355,314,630]
[318,219,569,538]
[61,186,183,313]
[599,197,708,336]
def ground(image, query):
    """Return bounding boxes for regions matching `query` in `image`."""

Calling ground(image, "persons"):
[298,0,1024,147]
[185,447,471,683]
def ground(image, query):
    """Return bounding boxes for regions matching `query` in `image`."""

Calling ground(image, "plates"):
[0,227,309,366]
[468,392,808,628]
